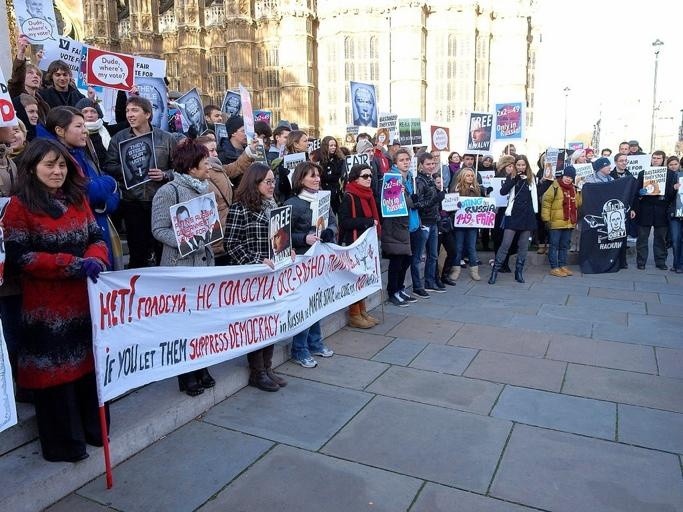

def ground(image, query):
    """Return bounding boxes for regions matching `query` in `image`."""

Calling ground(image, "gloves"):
[320,228,334,243]
[80,258,103,283]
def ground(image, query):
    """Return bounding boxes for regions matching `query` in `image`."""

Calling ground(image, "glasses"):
[264,179,276,184]
[359,173,374,180]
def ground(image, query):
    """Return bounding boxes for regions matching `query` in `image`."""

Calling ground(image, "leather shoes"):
[196,368,215,388]
[656,264,668,270]
[638,263,645,269]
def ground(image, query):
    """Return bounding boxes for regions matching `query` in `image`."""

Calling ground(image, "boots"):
[488,249,524,284]
[247,348,280,392]
[349,304,375,328]
[263,344,287,387]
[359,299,381,325]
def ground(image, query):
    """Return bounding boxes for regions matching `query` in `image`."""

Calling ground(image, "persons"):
[223,162,295,392]
[337,164,381,328]
[542,166,582,277]
[201,197,222,243]
[271,207,290,255]
[1,140,110,461]
[354,88,377,127]
[283,161,334,368]
[0,34,179,271]
[176,206,205,256]
[135,84,164,128]
[151,142,216,397]
[471,114,492,150]
[168,91,351,268]
[637,150,679,270]
[352,133,494,307]
[125,141,151,188]
[185,97,207,136]
[536,140,682,273]
[226,96,240,116]
[487,144,538,284]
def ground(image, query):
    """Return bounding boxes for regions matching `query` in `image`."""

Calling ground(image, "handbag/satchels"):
[500,215,505,230]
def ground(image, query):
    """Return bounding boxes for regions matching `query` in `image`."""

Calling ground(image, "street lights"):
[649,39,664,155]
[562,86,571,149]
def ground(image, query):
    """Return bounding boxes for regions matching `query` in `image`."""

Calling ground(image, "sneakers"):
[310,347,334,357]
[550,267,567,277]
[290,356,317,368]
[560,267,572,276]
[536,247,546,253]
[389,254,482,307]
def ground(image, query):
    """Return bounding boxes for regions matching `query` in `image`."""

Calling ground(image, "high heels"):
[178,371,204,396]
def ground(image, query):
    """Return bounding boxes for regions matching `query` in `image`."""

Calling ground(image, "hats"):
[225,116,245,139]
[496,155,515,172]
[563,166,575,180]
[74,98,103,119]
[628,141,638,145]
[356,139,373,156]
[592,157,611,173]
[19,93,39,109]
